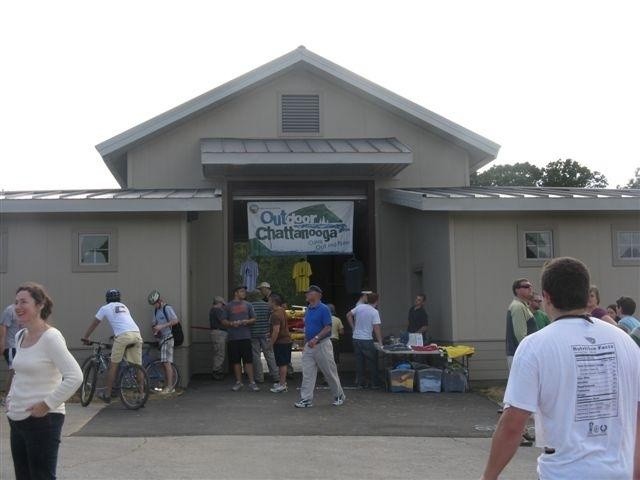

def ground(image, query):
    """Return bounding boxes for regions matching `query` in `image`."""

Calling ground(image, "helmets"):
[106,289,120,299]
[148,290,160,304]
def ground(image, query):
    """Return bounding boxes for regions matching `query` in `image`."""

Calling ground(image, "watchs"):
[315,336,319,340]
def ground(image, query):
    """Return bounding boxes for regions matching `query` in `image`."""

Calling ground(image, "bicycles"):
[109,336,180,394]
[80,338,150,410]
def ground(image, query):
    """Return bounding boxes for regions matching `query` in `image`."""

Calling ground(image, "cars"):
[286,305,307,317]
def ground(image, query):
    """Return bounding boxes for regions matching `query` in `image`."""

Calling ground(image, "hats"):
[233,284,248,289]
[303,285,322,293]
[361,288,373,294]
[255,282,270,290]
[215,296,226,305]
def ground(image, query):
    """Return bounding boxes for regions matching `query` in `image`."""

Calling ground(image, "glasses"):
[517,284,531,289]
[535,298,543,304]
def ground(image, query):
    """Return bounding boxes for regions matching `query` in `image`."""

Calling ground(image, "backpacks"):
[155,304,184,346]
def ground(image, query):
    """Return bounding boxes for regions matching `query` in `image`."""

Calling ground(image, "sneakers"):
[294,400,313,409]
[160,386,175,395]
[234,381,244,391]
[331,394,347,406]
[270,382,288,393]
[249,381,259,391]
[516,430,535,446]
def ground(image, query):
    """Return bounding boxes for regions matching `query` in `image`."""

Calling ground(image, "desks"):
[374,342,474,393]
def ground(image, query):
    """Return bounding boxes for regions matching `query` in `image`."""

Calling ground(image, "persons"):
[83,291,144,410]
[347,288,387,389]
[479,256,639,480]
[148,291,179,395]
[209,281,292,392]
[0,302,23,405]
[322,303,345,386]
[6,283,83,480]
[294,285,346,408]
[404,294,430,361]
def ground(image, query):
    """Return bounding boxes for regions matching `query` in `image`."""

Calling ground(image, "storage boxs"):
[442,368,468,393]
[387,367,415,393]
[414,364,443,393]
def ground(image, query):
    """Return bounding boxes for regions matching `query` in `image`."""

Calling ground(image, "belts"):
[213,327,227,332]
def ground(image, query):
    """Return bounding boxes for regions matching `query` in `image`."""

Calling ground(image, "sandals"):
[97,392,111,402]
